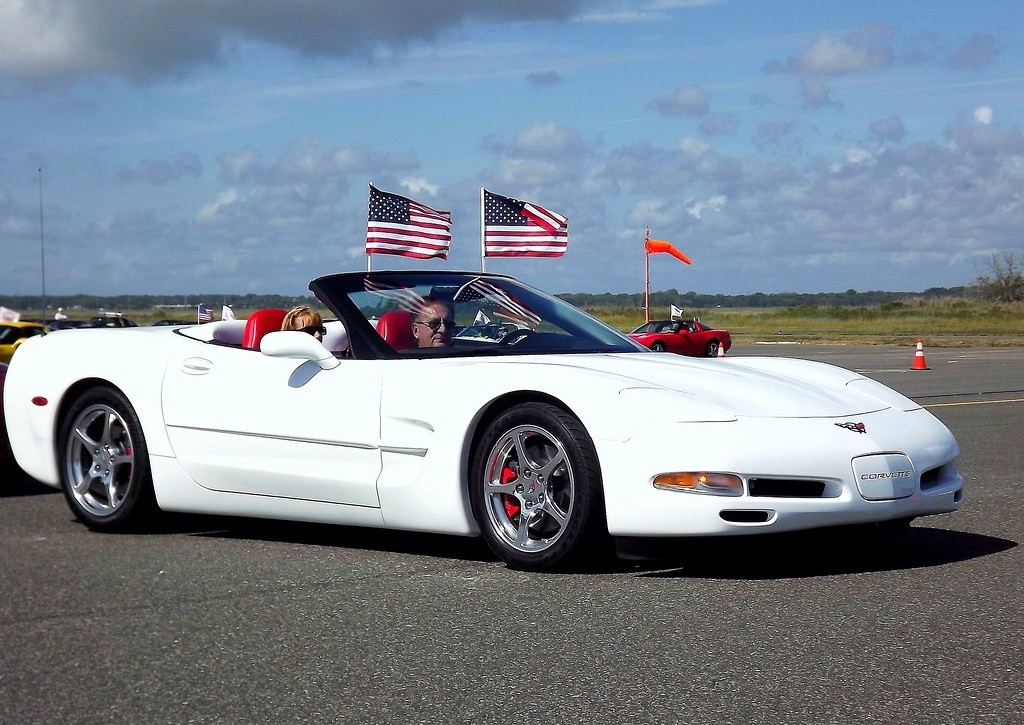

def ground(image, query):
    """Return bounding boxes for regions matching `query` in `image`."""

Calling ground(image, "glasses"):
[300,326,327,336]
[416,319,456,331]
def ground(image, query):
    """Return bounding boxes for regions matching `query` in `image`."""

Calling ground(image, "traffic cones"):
[909,337,931,371]
[717,342,726,358]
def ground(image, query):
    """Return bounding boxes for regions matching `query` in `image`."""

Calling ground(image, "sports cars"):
[4,269,968,572]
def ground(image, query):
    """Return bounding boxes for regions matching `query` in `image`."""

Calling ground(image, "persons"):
[408,296,456,348]
[55,307,68,321]
[280,304,327,344]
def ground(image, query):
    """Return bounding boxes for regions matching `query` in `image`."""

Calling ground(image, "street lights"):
[38,168,47,320]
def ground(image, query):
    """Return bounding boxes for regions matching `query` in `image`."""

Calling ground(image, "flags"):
[480,187,569,259]
[453,276,543,331]
[198,305,213,321]
[363,274,438,319]
[221,304,237,321]
[365,180,452,261]
[670,303,684,321]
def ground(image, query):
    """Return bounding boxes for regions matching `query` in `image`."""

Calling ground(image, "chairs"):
[376,310,417,351]
[241,308,288,349]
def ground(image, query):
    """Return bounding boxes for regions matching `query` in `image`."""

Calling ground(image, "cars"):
[174,321,209,325]
[0,321,57,364]
[627,320,732,357]
[89,317,139,328]
[140,319,189,327]
[48,321,97,331]
